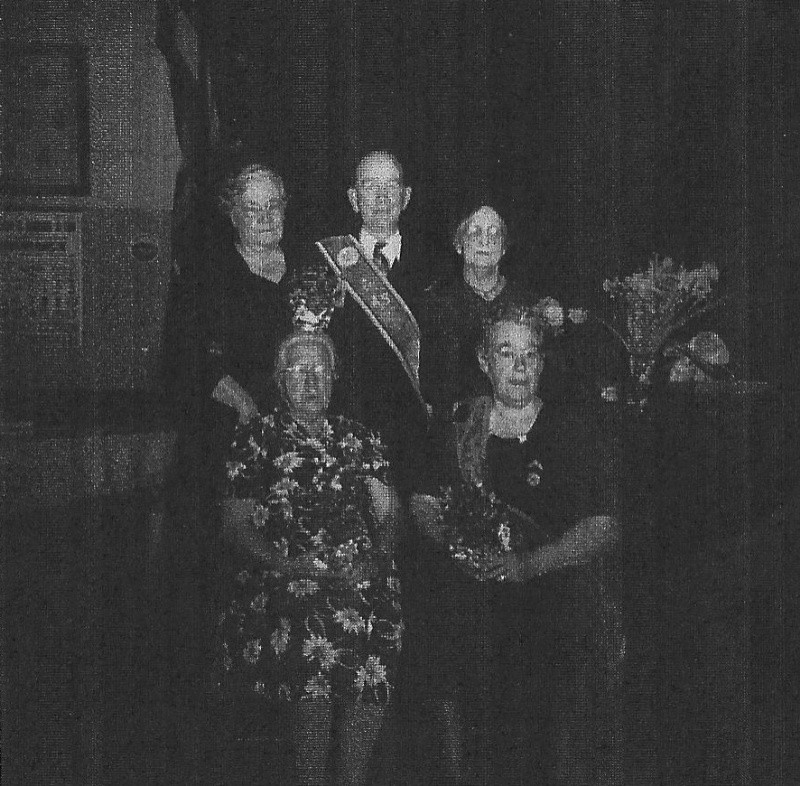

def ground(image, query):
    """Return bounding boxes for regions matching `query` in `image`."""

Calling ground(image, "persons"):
[415,194,594,435]
[215,331,402,784]
[193,161,336,434]
[289,149,433,435]
[410,303,626,785]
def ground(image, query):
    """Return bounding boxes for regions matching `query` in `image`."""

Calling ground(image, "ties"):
[371,241,391,277]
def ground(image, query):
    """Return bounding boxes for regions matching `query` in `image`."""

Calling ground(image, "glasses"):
[283,365,333,376]
[468,225,501,239]
